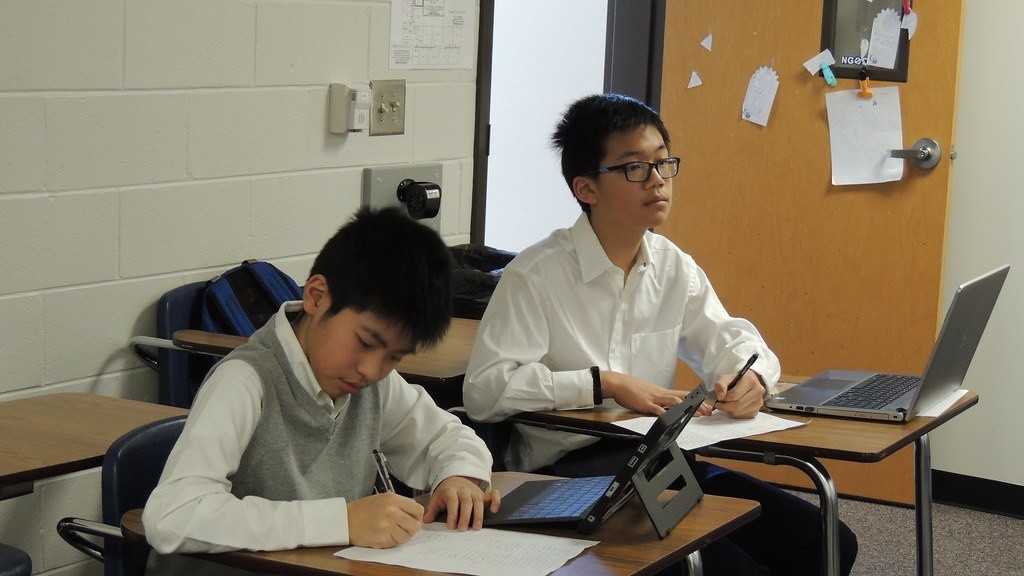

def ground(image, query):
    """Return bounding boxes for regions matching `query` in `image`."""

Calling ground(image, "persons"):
[462,91,859,576]
[141,205,500,576]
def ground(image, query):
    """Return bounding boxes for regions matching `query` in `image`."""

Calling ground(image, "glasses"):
[590,156,681,183]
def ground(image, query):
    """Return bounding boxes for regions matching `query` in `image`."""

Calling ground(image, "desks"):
[176,471,761,575]
[393,315,481,408]
[0,392,191,484]
[507,370,980,576]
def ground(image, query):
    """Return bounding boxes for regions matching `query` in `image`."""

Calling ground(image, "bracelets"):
[591,366,602,405]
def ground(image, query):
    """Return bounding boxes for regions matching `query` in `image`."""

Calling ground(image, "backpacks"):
[196,260,304,339]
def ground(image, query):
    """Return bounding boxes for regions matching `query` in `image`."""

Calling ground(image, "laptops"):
[463,384,708,532]
[764,264,1011,424]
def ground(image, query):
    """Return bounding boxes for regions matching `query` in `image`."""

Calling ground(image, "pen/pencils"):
[371,448,395,493]
[711,352,760,412]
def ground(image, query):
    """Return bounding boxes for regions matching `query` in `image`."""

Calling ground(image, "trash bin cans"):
[446,242,516,322]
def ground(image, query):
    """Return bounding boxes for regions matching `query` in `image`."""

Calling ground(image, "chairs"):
[128,280,250,409]
[57,415,188,576]
